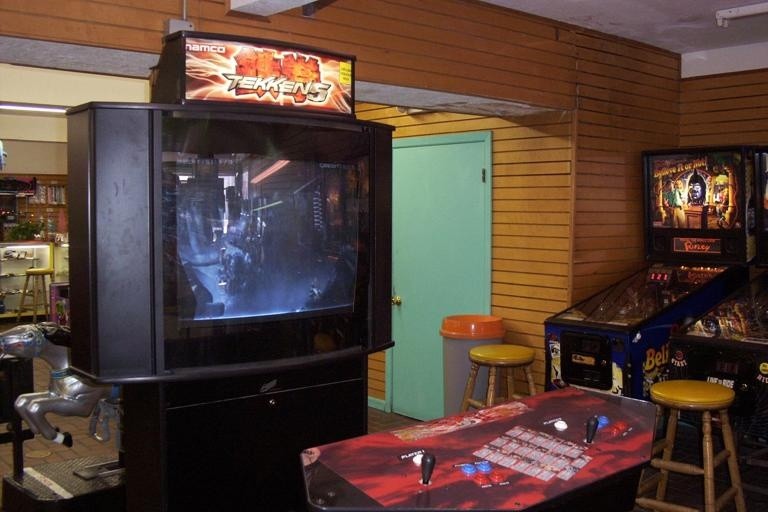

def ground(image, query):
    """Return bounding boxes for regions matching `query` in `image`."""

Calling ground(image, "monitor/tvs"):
[152,109,376,369]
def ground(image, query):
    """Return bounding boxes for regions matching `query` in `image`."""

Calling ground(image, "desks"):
[300,388,652,512]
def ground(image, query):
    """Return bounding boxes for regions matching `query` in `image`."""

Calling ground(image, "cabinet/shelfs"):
[0,174,71,320]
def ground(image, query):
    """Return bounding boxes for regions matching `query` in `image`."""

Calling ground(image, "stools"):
[460,343,535,412]
[634,380,746,512]
[17,268,56,323]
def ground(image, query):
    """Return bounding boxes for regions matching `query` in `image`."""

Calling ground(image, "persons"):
[663,180,687,227]
[179,175,333,290]
